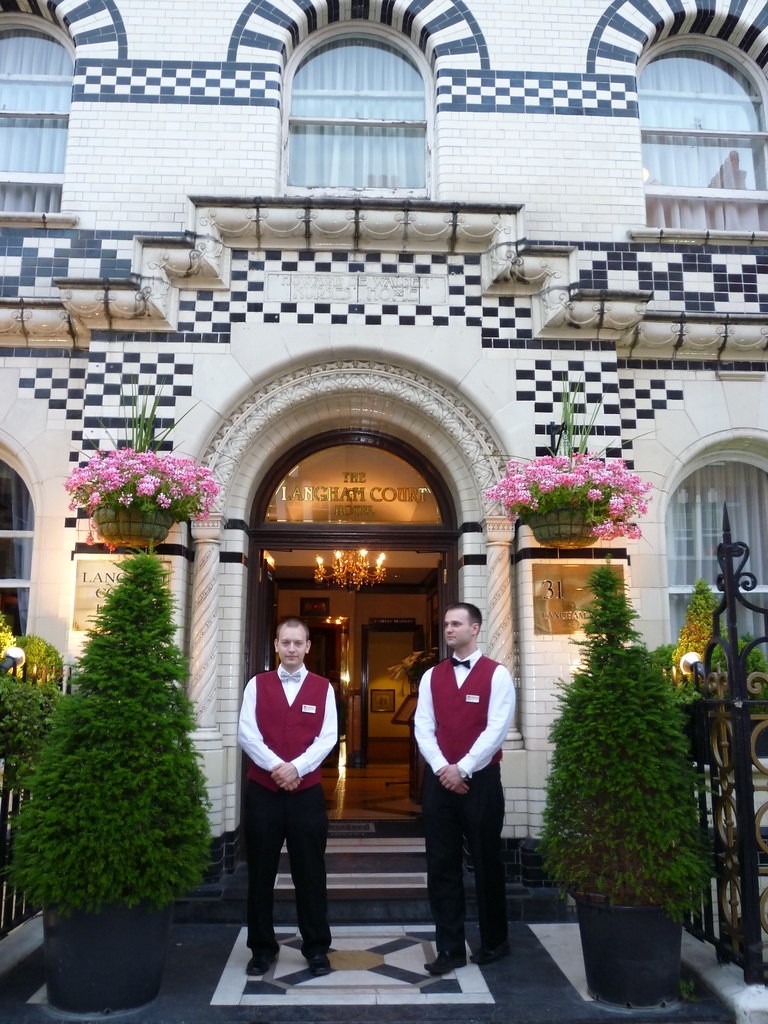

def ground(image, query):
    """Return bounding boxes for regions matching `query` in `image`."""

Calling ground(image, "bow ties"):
[452,658,471,668]
[280,671,301,682]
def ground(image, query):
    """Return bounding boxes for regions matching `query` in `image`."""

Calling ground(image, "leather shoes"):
[245,954,272,975]
[423,945,467,974]
[307,954,330,975]
[469,942,511,963]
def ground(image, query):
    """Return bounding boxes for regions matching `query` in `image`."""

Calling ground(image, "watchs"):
[457,765,468,781]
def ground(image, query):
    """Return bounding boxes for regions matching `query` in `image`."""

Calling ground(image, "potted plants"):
[535,561,721,1009]
[6,537,216,1015]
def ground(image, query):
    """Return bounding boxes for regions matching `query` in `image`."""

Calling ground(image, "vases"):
[93,503,174,547]
[522,501,599,547]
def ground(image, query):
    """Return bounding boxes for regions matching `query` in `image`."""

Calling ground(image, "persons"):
[236,618,339,980]
[413,602,517,974]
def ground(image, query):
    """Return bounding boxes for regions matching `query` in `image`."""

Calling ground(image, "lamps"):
[0,646,25,676]
[679,651,705,678]
[313,491,385,595]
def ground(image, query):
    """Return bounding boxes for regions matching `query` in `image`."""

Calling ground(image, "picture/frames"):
[370,689,396,713]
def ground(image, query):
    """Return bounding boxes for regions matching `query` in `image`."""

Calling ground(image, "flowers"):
[50,372,221,551]
[467,367,660,541]
